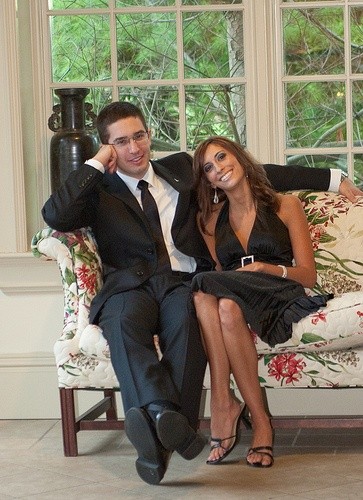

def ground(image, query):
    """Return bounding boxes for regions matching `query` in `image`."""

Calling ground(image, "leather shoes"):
[123,405,169,485]
[155,410,204,460]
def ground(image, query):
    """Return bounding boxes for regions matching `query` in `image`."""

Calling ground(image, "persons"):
[188,134,319,470]
[39,101,363,485]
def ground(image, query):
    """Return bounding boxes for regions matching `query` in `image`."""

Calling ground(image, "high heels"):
[246,427,275,468]
[205,401,252,464]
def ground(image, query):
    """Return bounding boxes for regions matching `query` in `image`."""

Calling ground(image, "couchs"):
[30,189,363,457]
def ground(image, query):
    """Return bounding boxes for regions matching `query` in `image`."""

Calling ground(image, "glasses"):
[107,131,148,147]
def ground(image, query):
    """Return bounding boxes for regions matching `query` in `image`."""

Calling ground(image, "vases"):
[46,85,100,198]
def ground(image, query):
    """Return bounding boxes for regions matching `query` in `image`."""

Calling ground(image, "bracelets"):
[341,170,348,182]
[276,263,288,278]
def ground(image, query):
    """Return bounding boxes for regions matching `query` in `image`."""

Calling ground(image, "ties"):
[137,180,172,271]
